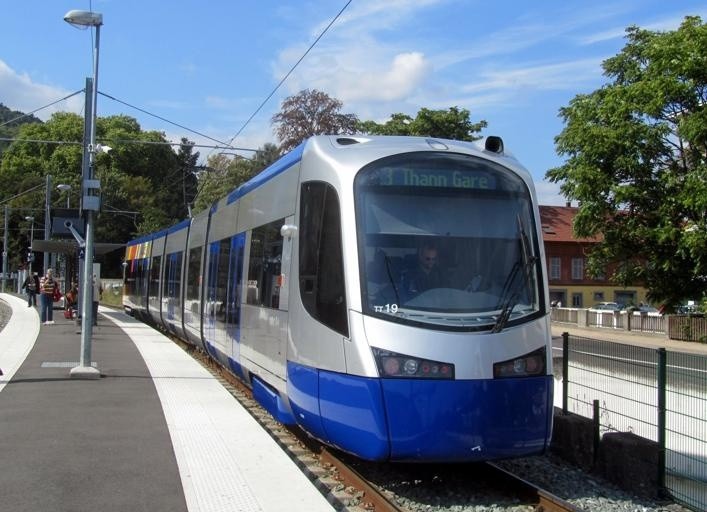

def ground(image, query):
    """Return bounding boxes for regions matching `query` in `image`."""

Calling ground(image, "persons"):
[22,271,40,307]
[40,269,58,325]
[91,275,103,327]
[401,242,452,301]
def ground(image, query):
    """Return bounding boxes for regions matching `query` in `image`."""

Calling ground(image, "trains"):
[121,132,554,466]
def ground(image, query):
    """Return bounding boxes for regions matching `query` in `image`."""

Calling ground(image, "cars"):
[585,301,698,315]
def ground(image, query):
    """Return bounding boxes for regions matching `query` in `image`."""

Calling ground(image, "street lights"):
[62,9,104,379]
[25,215,36,278]
[54,184,74,209]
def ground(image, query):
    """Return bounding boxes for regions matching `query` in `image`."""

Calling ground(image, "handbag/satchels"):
[54,282,60,302]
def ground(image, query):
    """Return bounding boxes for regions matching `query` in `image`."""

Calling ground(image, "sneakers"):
[46,320,54,324]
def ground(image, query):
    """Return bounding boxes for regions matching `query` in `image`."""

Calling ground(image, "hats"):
[48,269,53,274]
